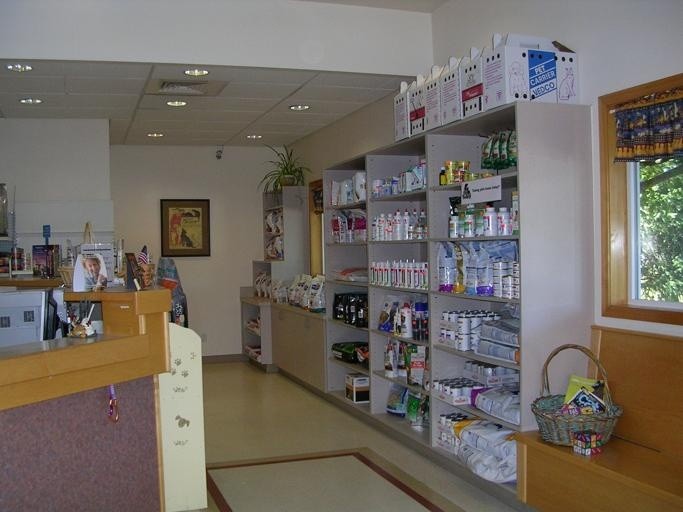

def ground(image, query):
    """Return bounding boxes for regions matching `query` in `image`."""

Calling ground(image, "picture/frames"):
[160,199,210,257]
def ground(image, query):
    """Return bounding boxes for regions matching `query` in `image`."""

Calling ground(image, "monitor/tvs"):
[41,287,69,342]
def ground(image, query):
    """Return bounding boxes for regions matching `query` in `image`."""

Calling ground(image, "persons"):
[81,254,107,292]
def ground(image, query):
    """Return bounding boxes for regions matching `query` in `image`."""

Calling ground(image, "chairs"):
[508,325,683,512]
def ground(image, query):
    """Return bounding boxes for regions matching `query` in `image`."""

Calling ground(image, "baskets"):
[532,343,623,446]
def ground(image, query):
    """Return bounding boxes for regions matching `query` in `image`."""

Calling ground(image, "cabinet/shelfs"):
[240,186,308,377]
[323,102,592,505]
[272,178,328,399]
[0,272,171,512]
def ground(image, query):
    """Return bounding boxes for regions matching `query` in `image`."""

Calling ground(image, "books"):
[564,376,608,405]
[558,389,609,415]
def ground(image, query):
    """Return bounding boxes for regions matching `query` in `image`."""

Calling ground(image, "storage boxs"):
[392,80,411,143]
[443,51,462,123]
[409,75,429,137]
[459,35,503,118]
[485,35,580,108]
[425,61,443,131]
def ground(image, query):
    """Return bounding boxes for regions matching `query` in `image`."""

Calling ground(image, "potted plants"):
[256,143,312,210]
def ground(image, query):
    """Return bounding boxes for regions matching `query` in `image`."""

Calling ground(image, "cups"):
[140,264,155,290]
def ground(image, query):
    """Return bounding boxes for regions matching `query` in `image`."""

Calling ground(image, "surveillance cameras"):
[216,150,222,160]
